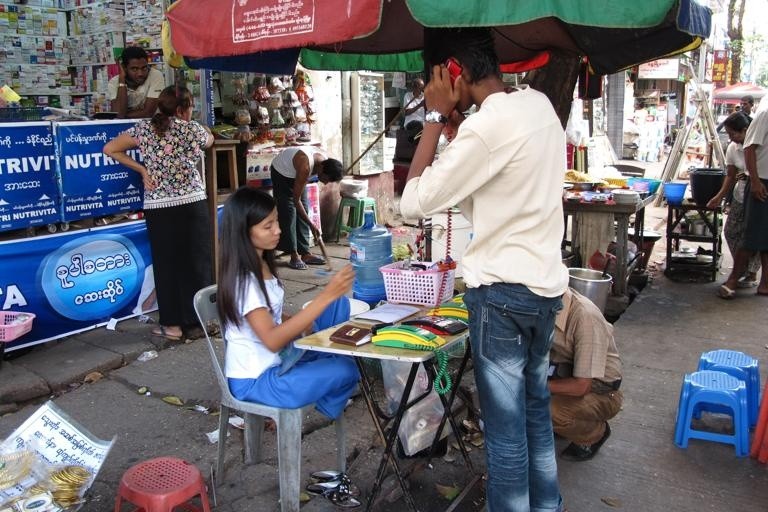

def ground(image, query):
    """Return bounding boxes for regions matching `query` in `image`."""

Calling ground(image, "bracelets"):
[117,83,128,87]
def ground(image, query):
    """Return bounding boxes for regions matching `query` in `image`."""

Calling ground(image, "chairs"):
[193,283,350,512]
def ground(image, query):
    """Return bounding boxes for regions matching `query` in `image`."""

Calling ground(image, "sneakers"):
[737,279,758,288]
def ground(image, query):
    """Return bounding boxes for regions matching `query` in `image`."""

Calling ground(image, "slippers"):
[151,327,180,340]
[716,282,736,299]
[289,254,325,269]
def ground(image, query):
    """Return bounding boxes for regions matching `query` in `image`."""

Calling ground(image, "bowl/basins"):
[628,176,663,194]
[567,196,582,204]
[688,221,709,236]
[631,190,649,200]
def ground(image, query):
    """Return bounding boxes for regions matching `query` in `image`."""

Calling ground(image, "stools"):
[114,455,210,511]
[335,196,377,242]
[694,350,760,429]
[673,369,750,458]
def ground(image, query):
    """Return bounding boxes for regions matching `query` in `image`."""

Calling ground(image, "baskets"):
[0,108,43,122]
[379,261,456,306]
[0,311,36,342]
[602,178,626,187]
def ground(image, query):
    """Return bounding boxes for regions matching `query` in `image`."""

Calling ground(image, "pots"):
[564,182,593,190]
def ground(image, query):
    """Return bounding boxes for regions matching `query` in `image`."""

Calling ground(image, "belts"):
[600,379,621,391]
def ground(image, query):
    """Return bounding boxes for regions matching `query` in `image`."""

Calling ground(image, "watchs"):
[422,110,450,125]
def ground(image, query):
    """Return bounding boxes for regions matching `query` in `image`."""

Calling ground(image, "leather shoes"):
[562,422,610,461]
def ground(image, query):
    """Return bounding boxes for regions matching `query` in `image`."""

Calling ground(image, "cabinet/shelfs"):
[1,0,175,118]
[665,202,723,281]
[350,71,400,176]
[623,88,678,160]
[203,138,241,286]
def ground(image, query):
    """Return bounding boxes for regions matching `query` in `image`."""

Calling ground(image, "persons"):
[707,96,768,299]
[399,27,568,512]
[547,288,623,460]
[106,48,164,117]
[102,85,215,341]
[217,186,361,420]
[403,78,427,141]
[270,145,345,271]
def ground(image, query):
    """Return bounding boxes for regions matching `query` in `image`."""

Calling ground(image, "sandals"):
[306,470,359,508]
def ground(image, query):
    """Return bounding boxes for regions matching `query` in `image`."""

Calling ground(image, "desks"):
[292,303,486,512]
[563,192,656,296]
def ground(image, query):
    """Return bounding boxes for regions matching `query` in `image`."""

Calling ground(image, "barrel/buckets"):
[348,210,393,300]
[663,182,688,206]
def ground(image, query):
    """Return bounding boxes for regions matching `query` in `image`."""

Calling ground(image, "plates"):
[611,189,641,206]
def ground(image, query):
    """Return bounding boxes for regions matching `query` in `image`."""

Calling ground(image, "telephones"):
[447,293,466,302]
[426,301,469,325]
[447,59,463,89]
[371,324,447,351]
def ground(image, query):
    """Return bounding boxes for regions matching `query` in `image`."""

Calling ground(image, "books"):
[329,324,372,347]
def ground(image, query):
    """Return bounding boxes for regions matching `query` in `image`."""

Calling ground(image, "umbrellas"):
[162,0,715,79]
